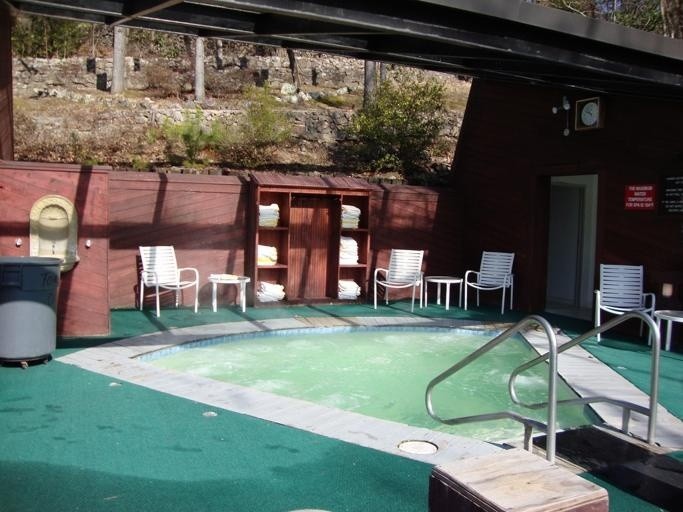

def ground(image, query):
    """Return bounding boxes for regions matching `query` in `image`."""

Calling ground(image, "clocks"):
[580,102,598,126]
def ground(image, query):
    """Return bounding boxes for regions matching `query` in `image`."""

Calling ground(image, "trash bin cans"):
[0,256,64,370]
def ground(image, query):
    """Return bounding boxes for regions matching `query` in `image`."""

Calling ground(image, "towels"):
[257,202,286,303]
[211,273,238,281]
[338,205,362,302]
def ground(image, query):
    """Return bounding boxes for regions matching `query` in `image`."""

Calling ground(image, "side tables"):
[653,309,683,350]
[424,275,463,311]
[207,276,251,314]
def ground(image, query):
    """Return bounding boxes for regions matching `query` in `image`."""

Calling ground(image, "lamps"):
[551,95,570,115]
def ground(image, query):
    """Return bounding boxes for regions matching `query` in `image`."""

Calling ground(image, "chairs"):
[373,248,425,313]
[593,263,656,347]
[137,244,200,318]
[463,249,516,315]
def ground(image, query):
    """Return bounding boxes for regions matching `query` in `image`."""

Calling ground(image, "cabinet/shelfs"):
[247,172,376,311]
